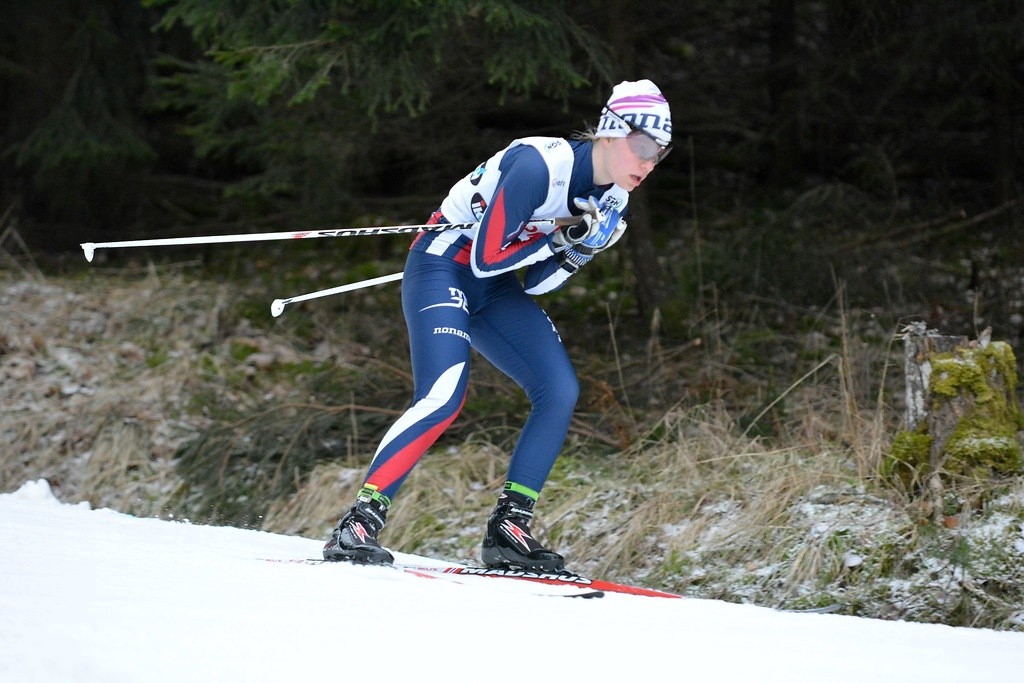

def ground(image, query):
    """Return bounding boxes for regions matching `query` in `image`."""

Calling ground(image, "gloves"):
[548,196,627,271]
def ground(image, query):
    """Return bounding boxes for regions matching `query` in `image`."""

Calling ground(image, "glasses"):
[627,129,673,165]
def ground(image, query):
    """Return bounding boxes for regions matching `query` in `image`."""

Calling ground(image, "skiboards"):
[246,553,842,614]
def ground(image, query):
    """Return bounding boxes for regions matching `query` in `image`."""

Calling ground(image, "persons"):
[324,79,672,570]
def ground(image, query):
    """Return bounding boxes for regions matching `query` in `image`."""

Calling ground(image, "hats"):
[595,79,672,139]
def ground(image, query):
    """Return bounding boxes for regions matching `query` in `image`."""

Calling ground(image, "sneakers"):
[323,501,394,565]
[481,492,565,573]
